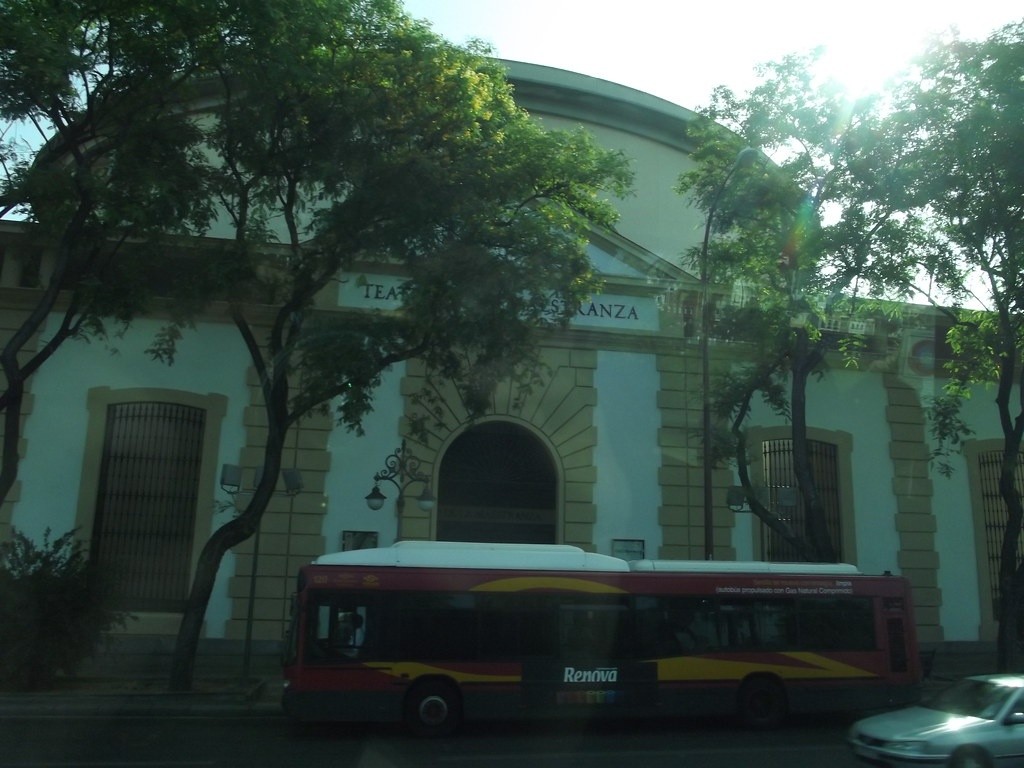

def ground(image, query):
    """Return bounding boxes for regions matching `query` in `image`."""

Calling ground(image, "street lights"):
[700,144,761,559]
[360,437,435,541]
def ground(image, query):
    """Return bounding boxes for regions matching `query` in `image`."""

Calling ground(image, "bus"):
[280,540,922,735]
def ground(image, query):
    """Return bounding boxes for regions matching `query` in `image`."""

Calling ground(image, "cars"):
[848,674,1024,768]
